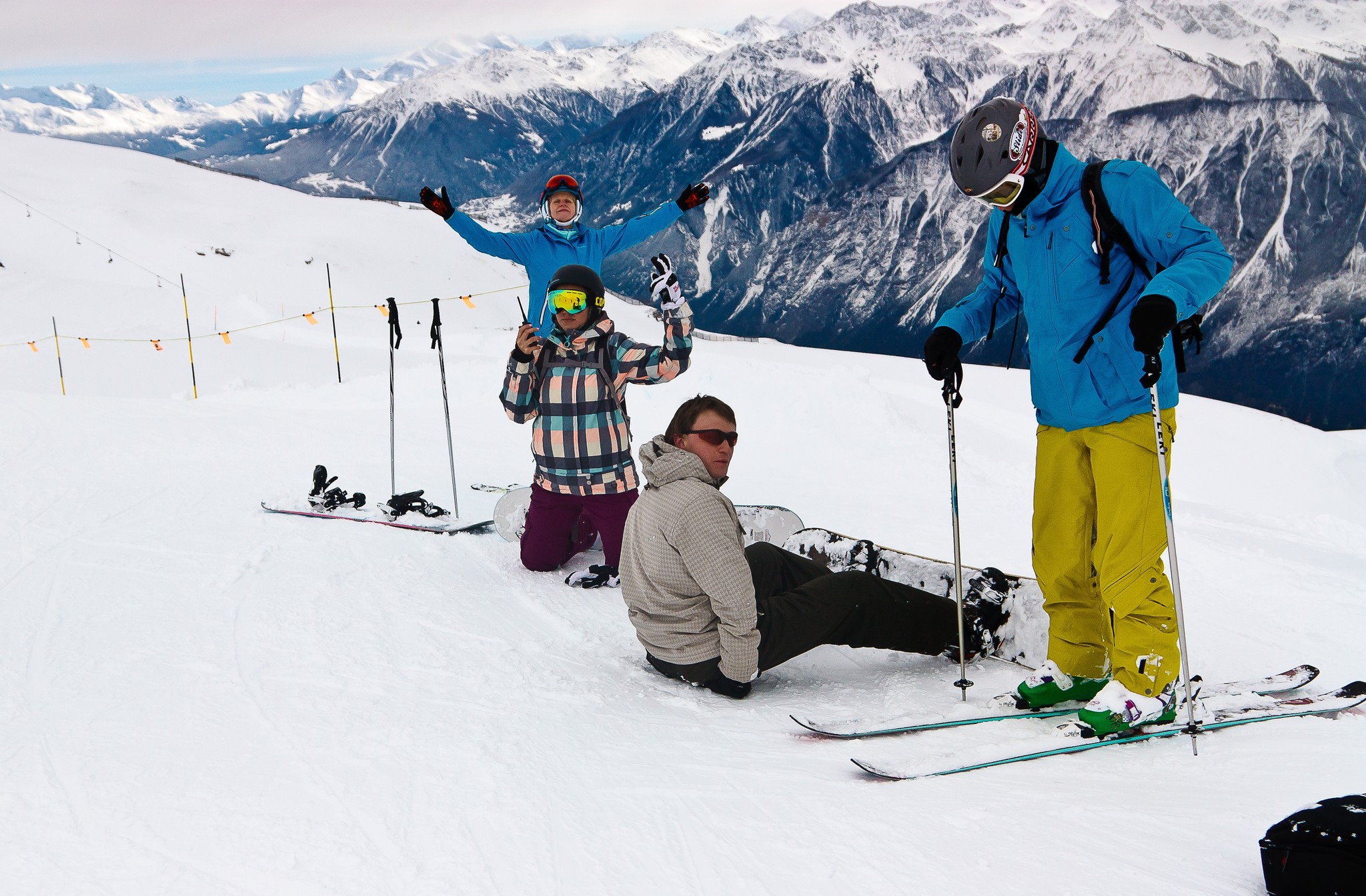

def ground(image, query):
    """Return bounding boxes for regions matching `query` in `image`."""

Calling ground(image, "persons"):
[922,98,1235,745]
[419,174,710,338]
[498,253,695,584]
[618,393,1013,700]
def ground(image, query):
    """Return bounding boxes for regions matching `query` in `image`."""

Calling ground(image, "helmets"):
[950,98,1047,196]
[539,184,582,224]
[548,264,605,319]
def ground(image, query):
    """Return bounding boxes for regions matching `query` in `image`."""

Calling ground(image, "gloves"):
[702,673,752,699]
[922,327,962,381]
[565,564,620,589]
[675,183,710,212]
[1128,294,1177,352]
[418,186,454,219]
[648,252,685,310]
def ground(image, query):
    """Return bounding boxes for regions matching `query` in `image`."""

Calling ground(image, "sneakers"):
[1077,680,1177,733]
[1017,672,1109,707]
[840,539,884,577]
[943,566,1013,664]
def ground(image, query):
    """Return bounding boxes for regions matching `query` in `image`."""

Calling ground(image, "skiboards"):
[470,482,531,493]
[788,663,1366,782]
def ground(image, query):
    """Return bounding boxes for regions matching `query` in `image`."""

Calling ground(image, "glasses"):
[683,429,738,447]
[970,173,1025,210]
[545,174,579,191]
[547,290,587,314]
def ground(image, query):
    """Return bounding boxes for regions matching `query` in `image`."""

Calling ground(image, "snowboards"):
[775,526,1063,668]
[493,488,808,554]
[261,492,494,534]
